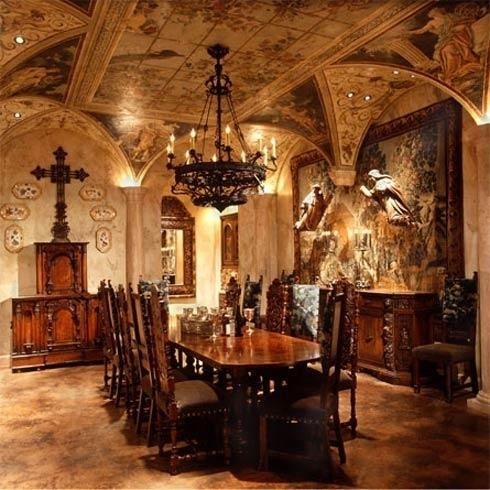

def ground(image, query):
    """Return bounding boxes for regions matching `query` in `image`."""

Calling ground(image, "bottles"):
[234,304,243,336]
[222,315,230,336]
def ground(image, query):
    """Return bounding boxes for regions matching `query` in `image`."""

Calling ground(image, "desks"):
[9,294,115,374]
[164,324,324,475]
[332,294,442,387]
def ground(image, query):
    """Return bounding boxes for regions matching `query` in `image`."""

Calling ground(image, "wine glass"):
[243,308,255,336]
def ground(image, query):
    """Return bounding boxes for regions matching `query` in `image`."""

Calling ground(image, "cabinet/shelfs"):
[218,213,238,310]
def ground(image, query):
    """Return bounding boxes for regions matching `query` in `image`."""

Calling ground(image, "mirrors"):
[159,217,197,298]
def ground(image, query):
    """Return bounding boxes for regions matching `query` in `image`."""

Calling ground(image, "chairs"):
[255,278,361,481]
[241,274,265,328]
[224,274,241,310]
[411,271,479,402]
[99,279,229,475]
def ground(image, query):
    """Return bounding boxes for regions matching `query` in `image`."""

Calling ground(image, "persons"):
[295,184,334,231]
[359,169,416,224]
[409,6,480,81]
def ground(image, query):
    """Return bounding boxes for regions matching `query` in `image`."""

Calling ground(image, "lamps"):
[162,44,278,213]
